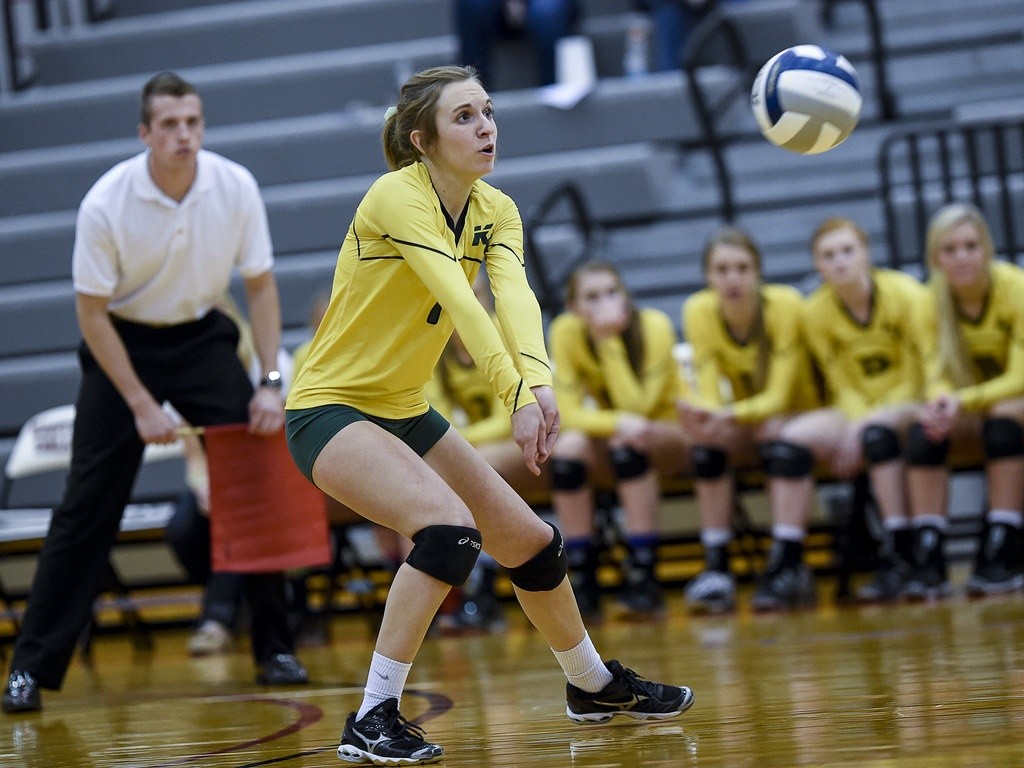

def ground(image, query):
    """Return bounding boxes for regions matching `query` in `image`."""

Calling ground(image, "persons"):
[284,66,694,767]
[0,73,308,712]
[165,200,1024,656]
[632,0,716,74]
[453,0,582,98]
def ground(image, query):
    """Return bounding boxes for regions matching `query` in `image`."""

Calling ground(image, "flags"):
[205,426,331,572]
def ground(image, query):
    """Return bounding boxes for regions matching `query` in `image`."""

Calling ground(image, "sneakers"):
[337,695,445,766]
[566,660,694,726]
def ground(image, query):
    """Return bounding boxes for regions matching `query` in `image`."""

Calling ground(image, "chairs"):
[0,405,94,670]
[106,401,198,651]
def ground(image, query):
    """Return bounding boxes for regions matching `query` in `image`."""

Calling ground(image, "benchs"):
[0,0,1024,592]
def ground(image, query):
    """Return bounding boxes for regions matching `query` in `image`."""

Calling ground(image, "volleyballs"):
[749,43,865,157]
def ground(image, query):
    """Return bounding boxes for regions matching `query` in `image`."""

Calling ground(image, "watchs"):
[260,370,282,388]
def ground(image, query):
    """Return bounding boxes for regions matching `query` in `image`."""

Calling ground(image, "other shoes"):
[454,589,501,631]
[569,568,600,614]
[904,567,950,601]
[751,573,814,612]
[255,653,309,683]
[189,630,230,653]
[1,685,42,716]
[965,563,1024,594]
[623,570,664,612]
[686,574,738,610]
[869,551,912,598]
